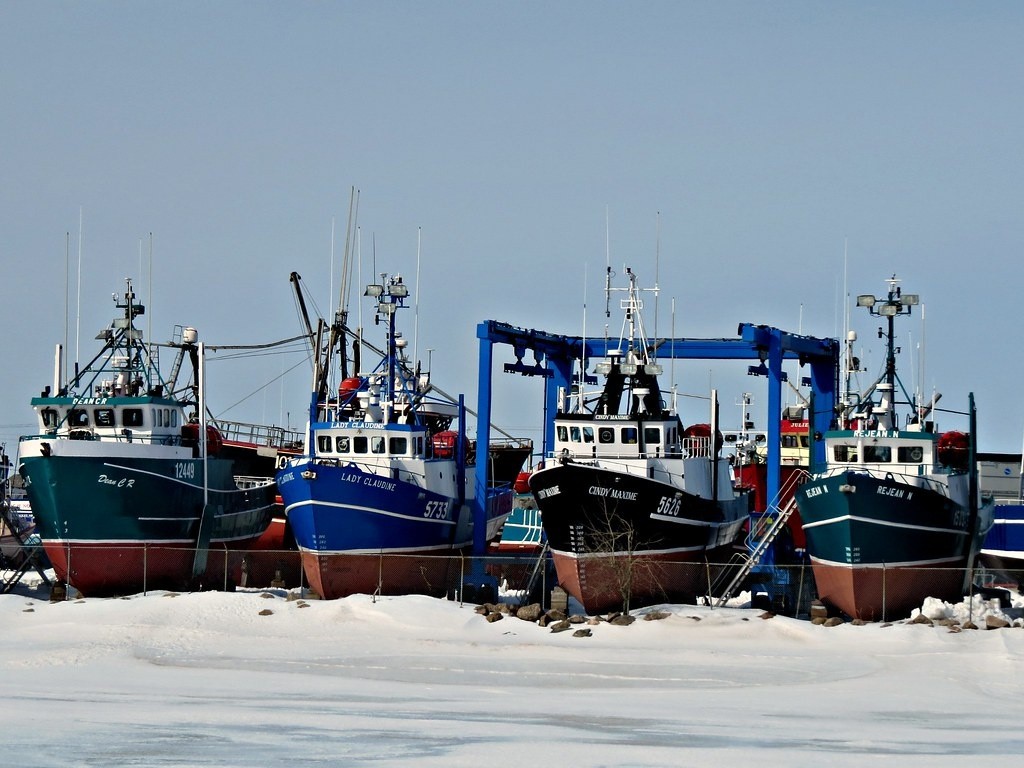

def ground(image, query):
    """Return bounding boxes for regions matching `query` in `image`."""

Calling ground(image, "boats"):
[2,205,304,600]
[278,187,535,600]
[729,235,1024,622]
[521,204,754,615]
